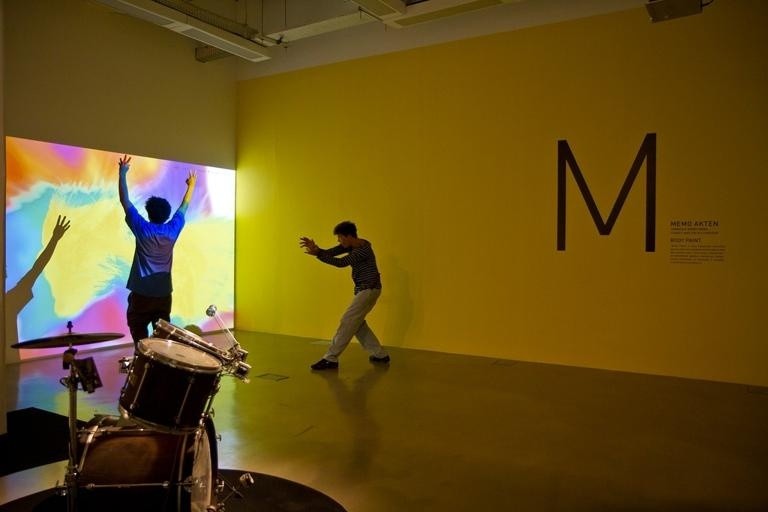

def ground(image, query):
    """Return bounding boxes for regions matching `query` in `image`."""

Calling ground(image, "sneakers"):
[311,358,338,370]
[369,355,390,362]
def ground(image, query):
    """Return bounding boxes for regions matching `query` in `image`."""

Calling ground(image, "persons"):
[301,220,390,370]
[117,154,198,349]
[5,214,71,364]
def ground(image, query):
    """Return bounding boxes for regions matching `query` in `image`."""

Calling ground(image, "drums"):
[118,338,223,436]
[62,413,220,511]
[150,318,228,365]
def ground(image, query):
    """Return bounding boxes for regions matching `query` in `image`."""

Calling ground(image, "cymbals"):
[9,332,125,349]
